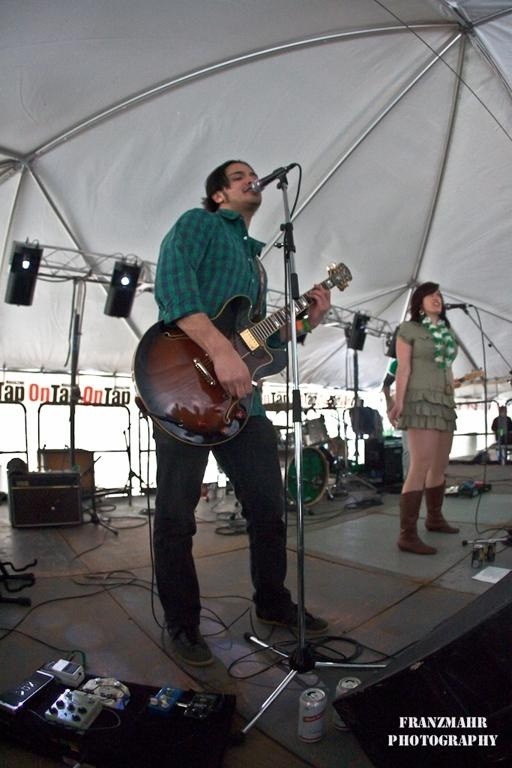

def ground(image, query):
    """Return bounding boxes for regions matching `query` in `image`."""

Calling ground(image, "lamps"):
[342,310,370,351]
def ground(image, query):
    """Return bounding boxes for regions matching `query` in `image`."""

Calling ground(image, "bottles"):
[332,677,364,731]
[299,688,328,743]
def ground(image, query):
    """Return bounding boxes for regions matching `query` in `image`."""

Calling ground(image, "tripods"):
[228,221,387,745]
[80,460,117,536]
[464,529,511,546]
[121,469,144,506]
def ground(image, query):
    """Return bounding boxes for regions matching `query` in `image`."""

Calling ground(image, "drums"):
[302,418,330,447]
[278,446,330,508]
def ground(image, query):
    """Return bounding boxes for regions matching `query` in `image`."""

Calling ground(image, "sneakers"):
[257,599,329,635]
[167,619,216,667]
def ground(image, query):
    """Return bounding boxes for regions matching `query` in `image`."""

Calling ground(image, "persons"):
[492,407,512,446]
[381,358,484,481]
[387,283,461,555]
[154,160,333,668]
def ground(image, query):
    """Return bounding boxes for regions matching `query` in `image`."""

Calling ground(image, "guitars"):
[133,263,352,448]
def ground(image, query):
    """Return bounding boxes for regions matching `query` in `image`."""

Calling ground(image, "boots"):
[425,483,461,535]
[396,490,439,555]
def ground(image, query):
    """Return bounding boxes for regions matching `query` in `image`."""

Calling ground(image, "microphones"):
[443,302,473,310]
[251,162,298,194]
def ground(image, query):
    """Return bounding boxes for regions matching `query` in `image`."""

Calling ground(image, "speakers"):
[364,437,406,484]
[6,470,80,528]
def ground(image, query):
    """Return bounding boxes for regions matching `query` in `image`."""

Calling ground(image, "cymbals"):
[264,403,293,411]
